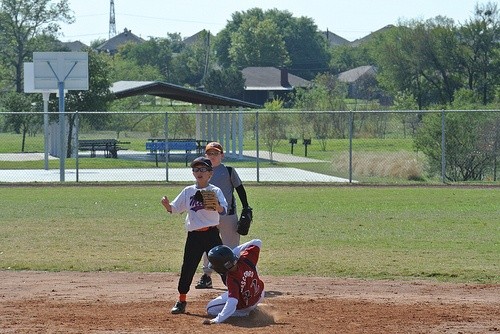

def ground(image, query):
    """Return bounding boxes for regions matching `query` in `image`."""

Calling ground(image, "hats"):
[190,157,214,171]
[205,142,223,152]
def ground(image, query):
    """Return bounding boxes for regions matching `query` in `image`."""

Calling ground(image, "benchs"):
[79,138,215,168]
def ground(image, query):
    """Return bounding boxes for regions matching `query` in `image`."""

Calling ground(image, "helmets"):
[207,245,239,273]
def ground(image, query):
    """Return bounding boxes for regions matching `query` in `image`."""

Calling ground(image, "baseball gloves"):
[193,189,220,211]
[235,206,254,236]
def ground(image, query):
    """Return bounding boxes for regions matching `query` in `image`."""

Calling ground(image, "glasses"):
[193,167,211,172]
[207,151,221,156]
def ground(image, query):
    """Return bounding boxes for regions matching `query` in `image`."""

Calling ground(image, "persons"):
[201,239,265,325]
[160,157,228,313]
[179,142,253,289]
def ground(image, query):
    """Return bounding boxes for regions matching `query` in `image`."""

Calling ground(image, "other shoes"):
[248,308,258,321]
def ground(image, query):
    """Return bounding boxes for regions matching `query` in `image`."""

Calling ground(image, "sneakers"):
[195,274,213,289]
[170,301,186,314]
